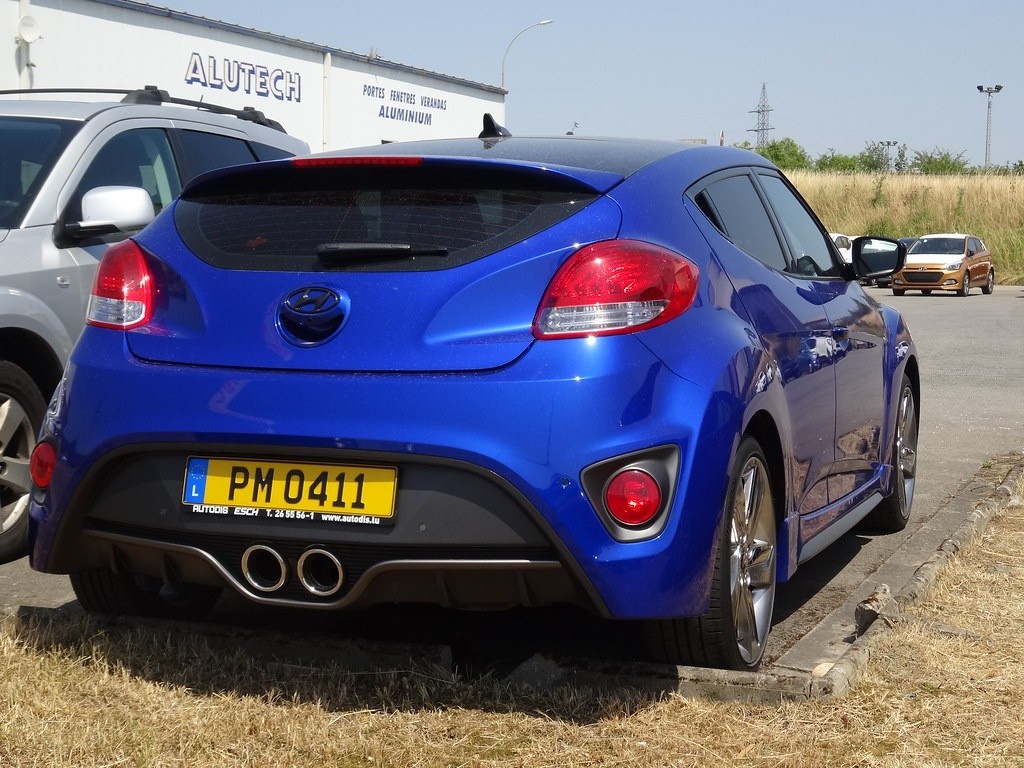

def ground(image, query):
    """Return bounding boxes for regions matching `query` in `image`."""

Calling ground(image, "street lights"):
[880,141,897,170]
[977,85,1003,174]
[501,20,553,87]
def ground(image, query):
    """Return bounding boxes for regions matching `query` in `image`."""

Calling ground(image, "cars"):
[828,232,995,297]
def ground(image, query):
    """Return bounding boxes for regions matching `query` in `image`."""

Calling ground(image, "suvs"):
[27,113,921,673]
[0,85,309,566]
